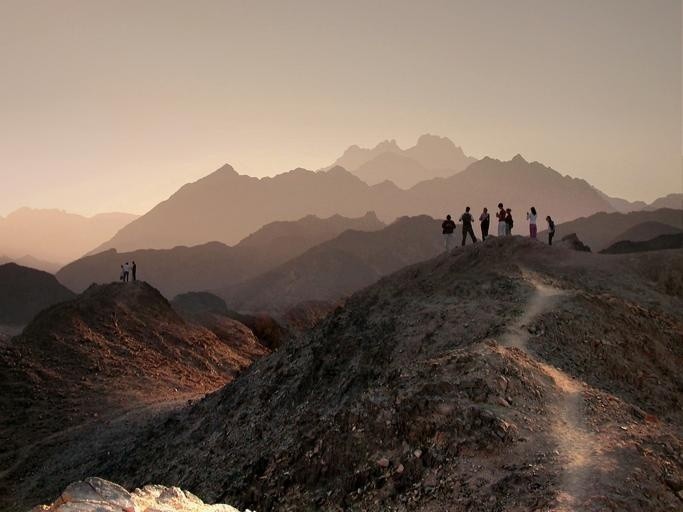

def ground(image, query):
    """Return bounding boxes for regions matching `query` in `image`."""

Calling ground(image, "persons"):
[130,260,136,281]
[122,262,130,282]
[504,208,513,236]
[495,203,506,237]
[478,207,490,242]
[119,265,123,282]
[545,215,555,246]
[526,206,537,240]
[440,214,456,253]
[458,206,477,247]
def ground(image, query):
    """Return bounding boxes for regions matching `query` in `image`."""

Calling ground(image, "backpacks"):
[445,224,453,233]
